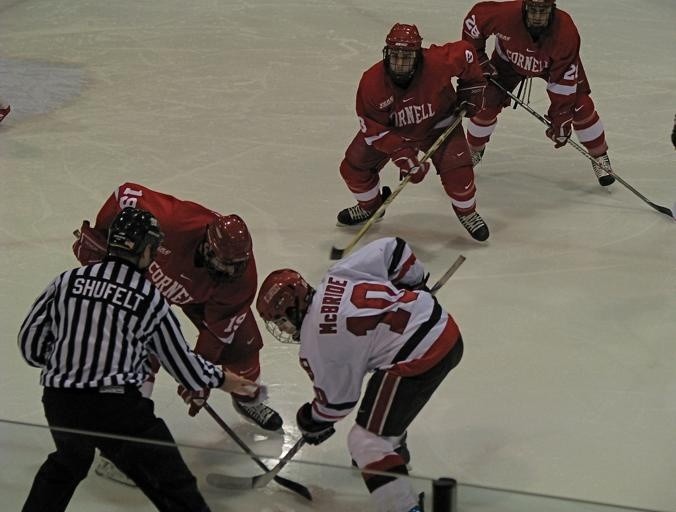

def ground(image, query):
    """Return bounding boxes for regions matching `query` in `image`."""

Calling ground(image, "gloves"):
[390,145,433,186]
[451,77,490,118]
[176,383,214,419]
[542,100,578,149]
[295,401,338,448]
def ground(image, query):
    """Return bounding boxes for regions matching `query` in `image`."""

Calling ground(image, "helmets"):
[254,266,317,322]
[104,206,164,258]
[385,24,424,51]
[524,0,556,9]
[204,214,254,268]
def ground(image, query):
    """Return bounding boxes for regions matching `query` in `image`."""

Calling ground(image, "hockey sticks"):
[202,401,311,499]
[306,109,467,261]
[488,76,674,217]
[208,256,466,491]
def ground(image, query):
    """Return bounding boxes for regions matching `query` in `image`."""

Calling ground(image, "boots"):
[452,204,491,243]
[336,186,391,226]
[592,152,617,188]
[230,396,284,432]
[468,144,486,169]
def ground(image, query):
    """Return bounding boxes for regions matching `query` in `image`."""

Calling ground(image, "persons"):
[461,0,616,186]
[336,21,490,244]
[16,206,259,511]
[71,181,283,431]
[255,236,466,512]
